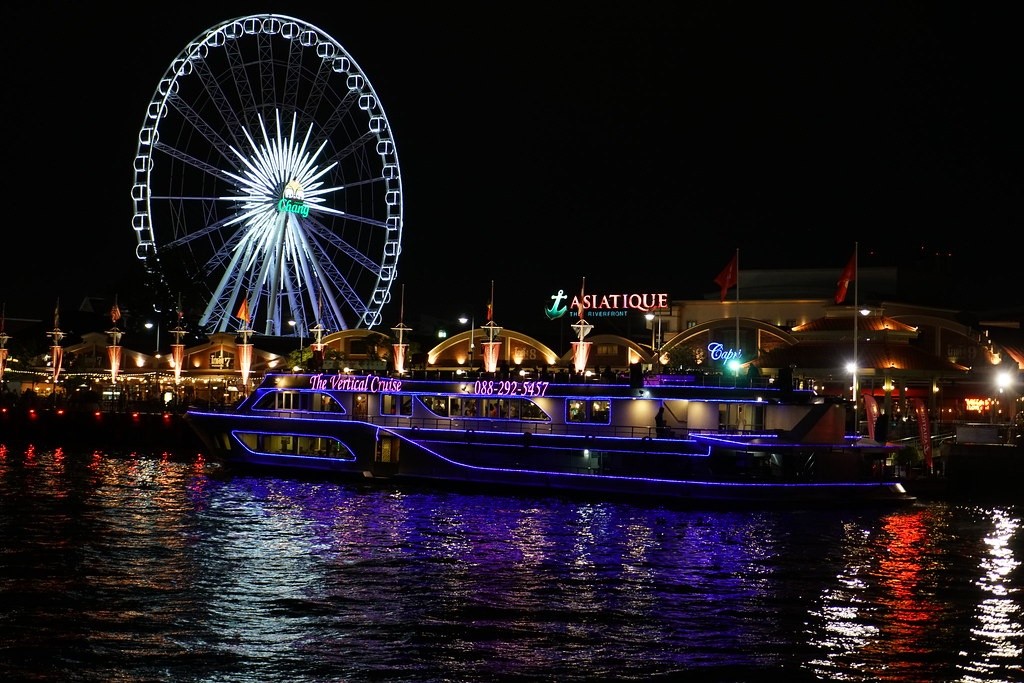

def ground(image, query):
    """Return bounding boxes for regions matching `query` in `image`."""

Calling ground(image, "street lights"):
[852,304,871,436]
[288,309,303,366]
[144,319,160,351]
[458,312,475,362]
[645,307,656,351]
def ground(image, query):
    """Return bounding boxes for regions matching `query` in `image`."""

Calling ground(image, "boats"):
[183,362,918,510]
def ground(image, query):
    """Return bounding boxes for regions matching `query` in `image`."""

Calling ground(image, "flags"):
[486,304,492,321]
[236,298,251,323]
[833,257,856,305]
[714,256,738,303]
[577,286,586,320]
[111,303,122,324]
[178,310,184,321]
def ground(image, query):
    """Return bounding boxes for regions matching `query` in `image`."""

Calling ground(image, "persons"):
[451,402,462,416]
[486,403,506,418]
[654,407,675,439]
